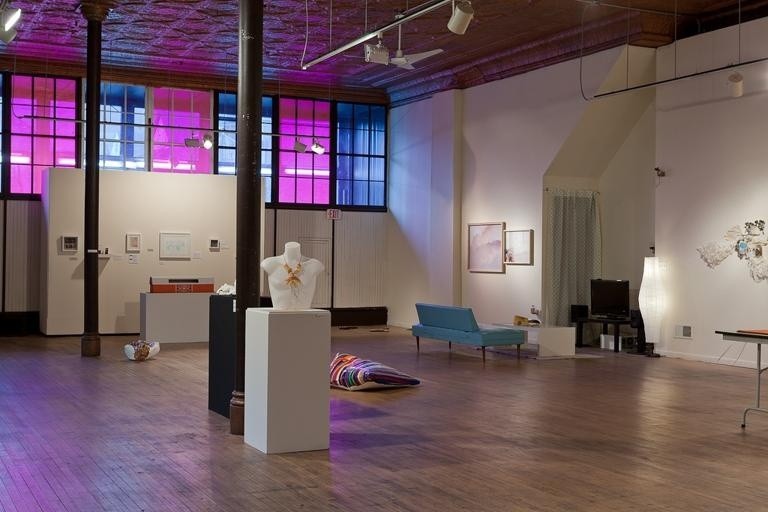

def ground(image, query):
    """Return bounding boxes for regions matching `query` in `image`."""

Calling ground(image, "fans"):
[343,14,445,70]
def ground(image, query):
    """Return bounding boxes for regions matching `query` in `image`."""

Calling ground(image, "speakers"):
[631,310,644,328]
[571,305,588,322]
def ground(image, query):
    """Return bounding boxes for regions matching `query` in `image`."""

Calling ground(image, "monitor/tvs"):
[591,279,629,320]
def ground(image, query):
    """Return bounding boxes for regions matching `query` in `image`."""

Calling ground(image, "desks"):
[714,330,768,428]
[576,316,644,353]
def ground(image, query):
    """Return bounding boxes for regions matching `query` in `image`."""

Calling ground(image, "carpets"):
[471,344,605,360]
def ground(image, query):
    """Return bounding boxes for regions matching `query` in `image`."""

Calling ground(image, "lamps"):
[726,70,744,98]
[0,5,21,44]
[364,33,389,66]
[184,129,200,147]
[293,136,307,153]
[311,137,325,155]
[202,130,213,150]
[447,0,474,36]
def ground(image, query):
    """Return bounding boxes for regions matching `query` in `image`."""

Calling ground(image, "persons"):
[260,241,326,312]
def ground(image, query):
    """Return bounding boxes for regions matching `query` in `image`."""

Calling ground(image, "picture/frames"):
[503,229,534,265]
[466,222,505,273]
[126,233,140,253]
[158,232,194,260]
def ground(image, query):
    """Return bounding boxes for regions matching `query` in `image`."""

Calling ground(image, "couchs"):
[412,302,525,363]
